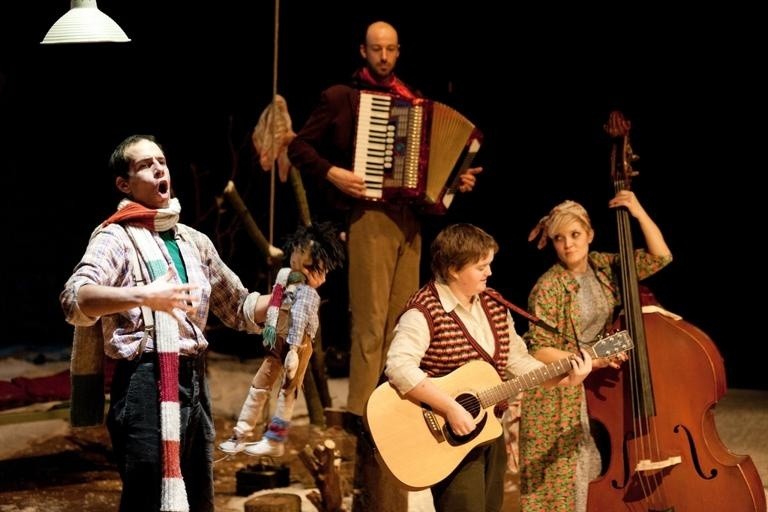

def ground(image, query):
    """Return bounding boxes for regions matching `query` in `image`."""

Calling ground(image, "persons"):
[381,224,595,511]
[55,133,331,509]
[504,189,675,512]
[284,20,488,439]
[218,220,346,460]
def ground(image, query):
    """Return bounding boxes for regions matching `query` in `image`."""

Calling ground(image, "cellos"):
[583,112,767,512]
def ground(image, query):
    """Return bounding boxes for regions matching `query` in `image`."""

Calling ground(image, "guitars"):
[362,328,635,491]
